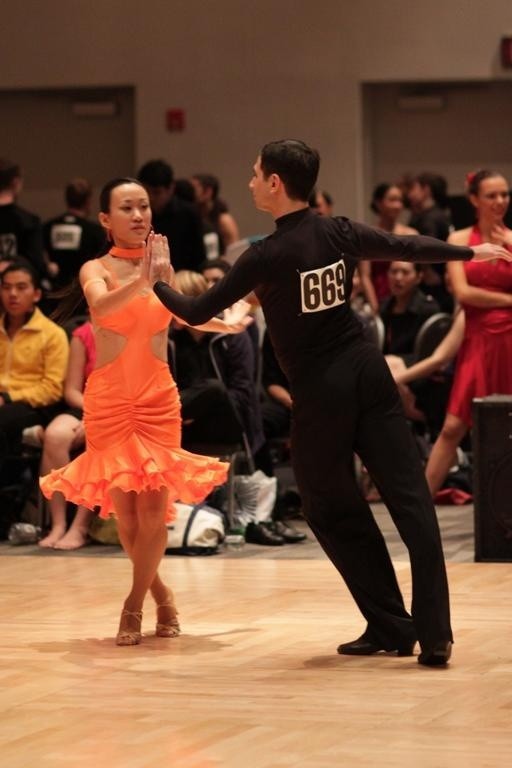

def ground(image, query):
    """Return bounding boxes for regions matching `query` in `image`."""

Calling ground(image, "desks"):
[470,391,512,564]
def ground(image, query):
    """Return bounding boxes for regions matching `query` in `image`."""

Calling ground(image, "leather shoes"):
[259,520,306,543]
[423,638,452,665]
[338,633,417,657]
[245,522,284,545]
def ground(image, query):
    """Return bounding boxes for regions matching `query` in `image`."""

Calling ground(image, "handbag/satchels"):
[167,498,225,556]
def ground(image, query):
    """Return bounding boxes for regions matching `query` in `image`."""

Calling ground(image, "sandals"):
[116,608,142,645]
[155,584,179,637]
